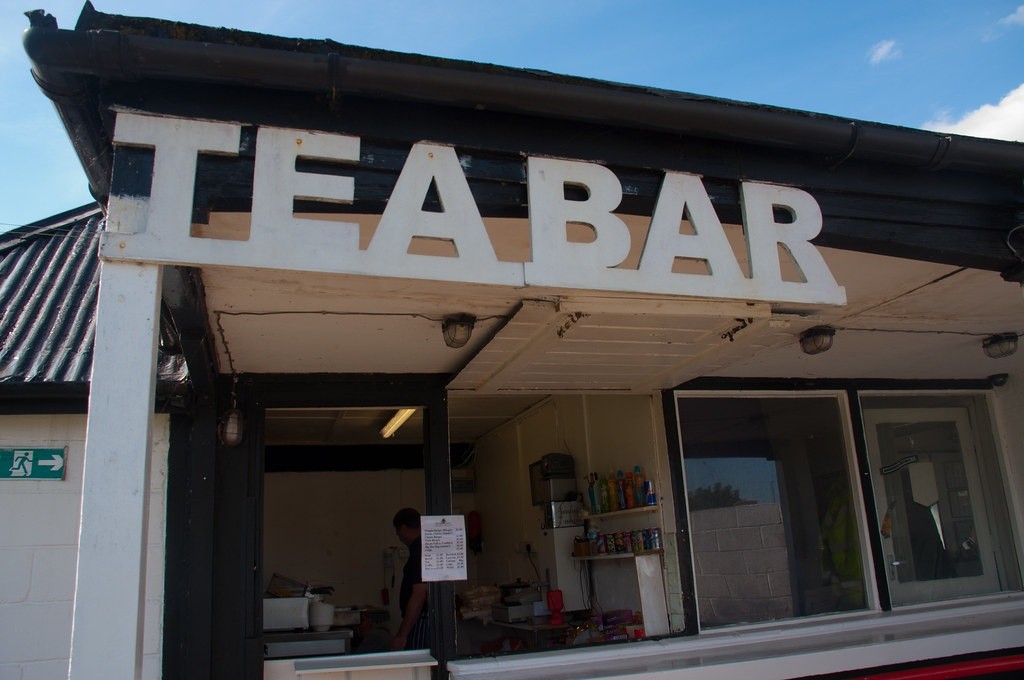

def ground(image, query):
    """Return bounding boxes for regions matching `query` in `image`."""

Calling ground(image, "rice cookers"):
[500,578,531,605]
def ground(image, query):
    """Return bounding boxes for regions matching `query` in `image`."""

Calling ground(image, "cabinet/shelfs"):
[571,506,669,639]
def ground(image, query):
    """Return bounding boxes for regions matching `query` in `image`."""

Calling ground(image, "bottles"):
[599,465,656,513]
[612,634,627,640]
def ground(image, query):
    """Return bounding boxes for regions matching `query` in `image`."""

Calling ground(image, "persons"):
[388,508,433,651]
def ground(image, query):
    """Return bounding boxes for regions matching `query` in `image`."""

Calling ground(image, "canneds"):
[643,480,656,505]
[597,526,661,556]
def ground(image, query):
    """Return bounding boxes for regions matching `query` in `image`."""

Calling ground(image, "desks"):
[490,618,571,651]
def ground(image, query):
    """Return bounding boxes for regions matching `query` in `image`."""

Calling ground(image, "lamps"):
[983,333,1018,359]
[220,405,244,447]
[799,328,837,355]
[380,408,415,439]
[442,315,478,349]
